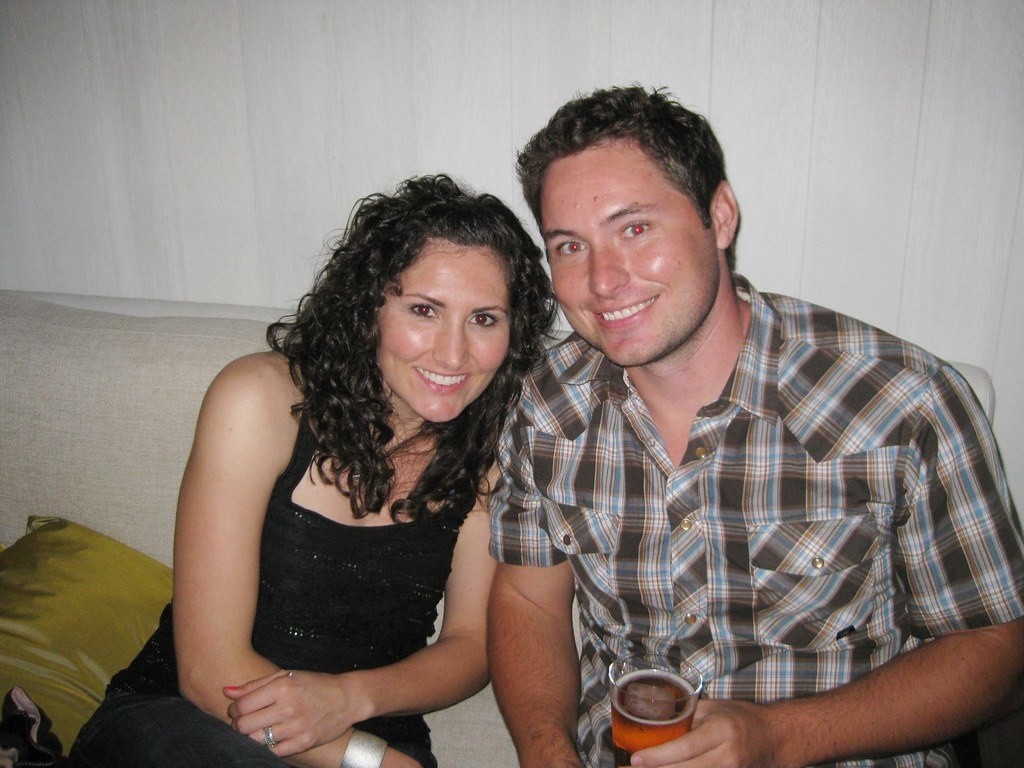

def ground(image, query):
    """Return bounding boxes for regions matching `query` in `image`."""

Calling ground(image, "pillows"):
[0,511,173,757]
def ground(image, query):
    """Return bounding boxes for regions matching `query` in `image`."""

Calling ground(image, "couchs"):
[0,290,995,768]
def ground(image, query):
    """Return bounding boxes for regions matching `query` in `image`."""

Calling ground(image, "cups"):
[609,651,704,767]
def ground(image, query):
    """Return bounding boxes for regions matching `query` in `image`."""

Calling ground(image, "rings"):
[262,726,277,748]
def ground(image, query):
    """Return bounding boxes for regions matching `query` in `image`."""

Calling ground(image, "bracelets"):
[339,730,388,768]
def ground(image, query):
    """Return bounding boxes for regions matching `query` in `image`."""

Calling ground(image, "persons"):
[488,87,1024,768]
[67,175,555,768]
